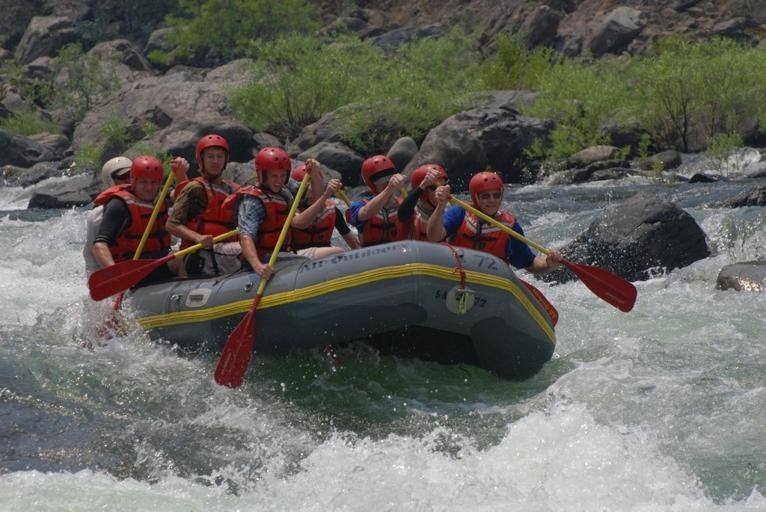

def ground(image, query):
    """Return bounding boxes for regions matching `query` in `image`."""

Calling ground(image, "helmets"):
[130,156,164,191]
[292,164,324,184]
[101,156,132,187]
[255,147,292,186]
[469,171,505,209]
[195,134,230,173]
[411,163,449,202]
[361,155,396,195]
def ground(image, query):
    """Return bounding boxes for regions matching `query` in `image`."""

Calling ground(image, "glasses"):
[481,193,502,199]
[427,184,448,191]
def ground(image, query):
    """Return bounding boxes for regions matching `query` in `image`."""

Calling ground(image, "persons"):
[344,154,417,248]
[398,163,456,246]
[165,134,254,279]
[83,157,180,289]
[425,171,563,274]
[290,163,360,256]
[94,156,190,295]
[232,146,344,272]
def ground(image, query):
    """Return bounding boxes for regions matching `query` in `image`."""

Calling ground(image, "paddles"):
[215,168,311,387]
[89,227,240,301]
[98,161,179,341]
[434,179,559,326]
[447,196,637,313]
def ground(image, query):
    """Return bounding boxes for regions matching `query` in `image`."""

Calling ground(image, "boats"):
[122,240,558,381]
